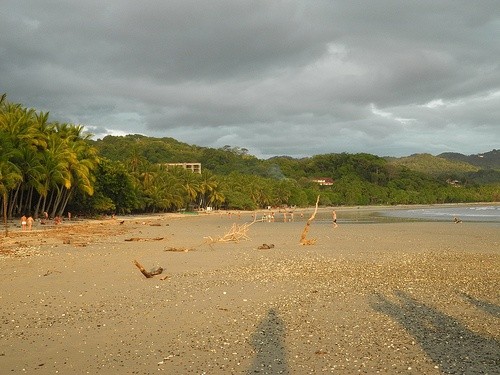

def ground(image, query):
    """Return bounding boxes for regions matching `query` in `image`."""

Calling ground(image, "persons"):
[21,215,26,231]
[454,217,461,224]
[40,211,115,226]
[229,210,305,222]
[331,211,338,228]
[26,215,34,231]
